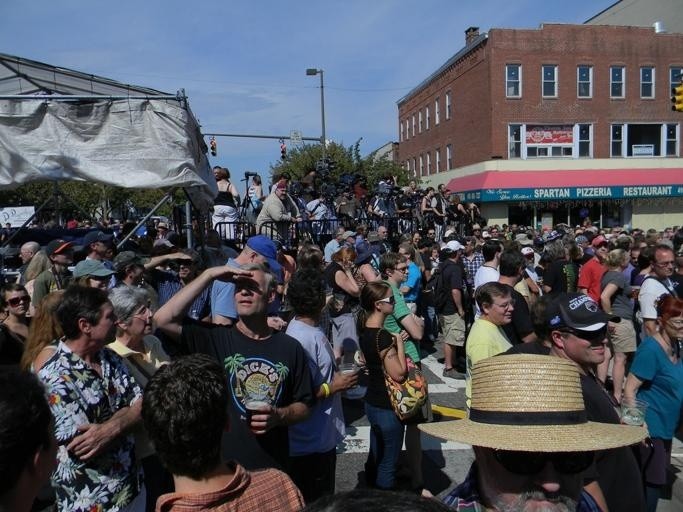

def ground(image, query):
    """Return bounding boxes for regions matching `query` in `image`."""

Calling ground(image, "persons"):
[114,210,179,249]
[16,218,115,239]
[465,282,514,419]
[0,371,57,512]
[299,244,322,268]
[544,240,578,300]
[18,242,40,282]
[104,286,152,358]
[254,182,297,240]
[377,226,392,253]
[213,166,222,181]
[297,164,377,243]
[141,353,306,512]
[398,244,422,314]
[638,244,678,340]
[418,353,649,512]
[377,165,486,226]
[354,242,382,287]
[625,293,683,512]
[399,226,485,255]
[36,286,144,512]
[1,223,15,248]
[32,239,74,310]
[417,238,435,281]
[243,176,266,222]
[326,248,362,367]
[210,236,282,325]
[271,172,290,195]
[358,281,408,490]
[285,268,358,503]
[112,251,145,287]
[0,283,35,371]
[436,240,466,380]
[273,240,296,283]
[211,168,241,239]
[324,226,344,268]
[71,259,118,292]
[378,254,424,372]
[462,237,485,288]
[486,218,588,263]
[597,248,637,406]
[23,252,50,280]
[524,247,543,294]
[144,248,212,324]
[548,293,644,512]
[474,240,504,322]
[21,289,66,374]
[497,249,537,346]
[588,218,683,266]
[82,231,114,260]
[152,263,318,473]
[578,236,610,303]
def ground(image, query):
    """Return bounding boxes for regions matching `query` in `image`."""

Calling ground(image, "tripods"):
[239,181,258,221]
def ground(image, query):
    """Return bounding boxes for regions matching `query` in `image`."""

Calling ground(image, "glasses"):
[650,262,676,269]
[483,450,596,475]
[590,235,607,248]
[374,295,394,304]
[474,230,480,232]
[6,294,32,307]
[394,265,409,273]
[427,233,436,235]
[128,308,155,320]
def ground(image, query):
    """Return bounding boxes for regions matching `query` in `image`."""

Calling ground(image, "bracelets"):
[323,383,330,398]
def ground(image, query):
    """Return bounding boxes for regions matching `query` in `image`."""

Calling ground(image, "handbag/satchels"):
[375,328,429,421]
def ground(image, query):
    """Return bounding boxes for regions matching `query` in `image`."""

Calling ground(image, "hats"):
[444,241,466,254]
[44,238,76,259]
[82,230,115,247]
[150,238,174,253]
[343,230,357,240]
[417,353,650,453]
[366,231,382,244]
[246,234,281,271]
[71,259,116,282]
[157,222,168,229]
[541,291,621,333]
[472,224,481,230]
[354,241,375,264]
[514,234,535,246]
[441,230,453,238]
[543,230,562,241]
[111,251,152,272]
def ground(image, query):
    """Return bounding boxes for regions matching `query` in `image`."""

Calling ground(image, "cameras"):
[244,171,258,179]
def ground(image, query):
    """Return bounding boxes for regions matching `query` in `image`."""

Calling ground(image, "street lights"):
[307,67,326,143]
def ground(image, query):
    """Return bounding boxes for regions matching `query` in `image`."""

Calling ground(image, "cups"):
[244,394,271,434]
[620,397,649,429]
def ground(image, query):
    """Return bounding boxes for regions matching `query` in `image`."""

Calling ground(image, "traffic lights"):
[671,87,683,111]
[281,145,286,161]
[210,140,217,157]
